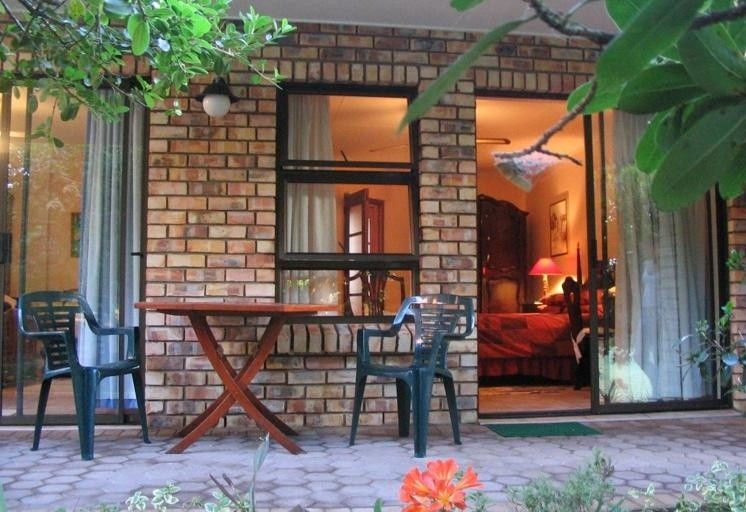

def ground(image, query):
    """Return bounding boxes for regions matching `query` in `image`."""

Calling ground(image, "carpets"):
[485,420,601,439]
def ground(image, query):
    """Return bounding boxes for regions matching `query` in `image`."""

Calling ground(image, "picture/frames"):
[69,211,80,259]
[547,199,568,257]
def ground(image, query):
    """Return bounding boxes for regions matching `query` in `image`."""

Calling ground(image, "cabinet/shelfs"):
[477,194,530,314]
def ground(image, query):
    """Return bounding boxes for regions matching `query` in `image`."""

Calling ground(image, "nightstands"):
[520,302,546,314]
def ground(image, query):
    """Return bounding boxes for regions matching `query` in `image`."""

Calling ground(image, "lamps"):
[527,257,562,296]
[192,77,239,120]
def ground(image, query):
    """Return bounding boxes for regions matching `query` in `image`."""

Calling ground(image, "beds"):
[477,242,603,382]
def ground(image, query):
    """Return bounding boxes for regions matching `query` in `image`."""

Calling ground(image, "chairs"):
[16,290,151,461]
[349,291,474,460]
[560,276,591,390]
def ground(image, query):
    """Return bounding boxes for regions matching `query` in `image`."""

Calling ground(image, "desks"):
[131,301,342,456]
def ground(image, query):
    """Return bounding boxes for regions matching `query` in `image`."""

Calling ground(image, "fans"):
[476,136,510,147]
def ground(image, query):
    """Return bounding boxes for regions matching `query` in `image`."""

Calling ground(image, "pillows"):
[537,288,602,315]
